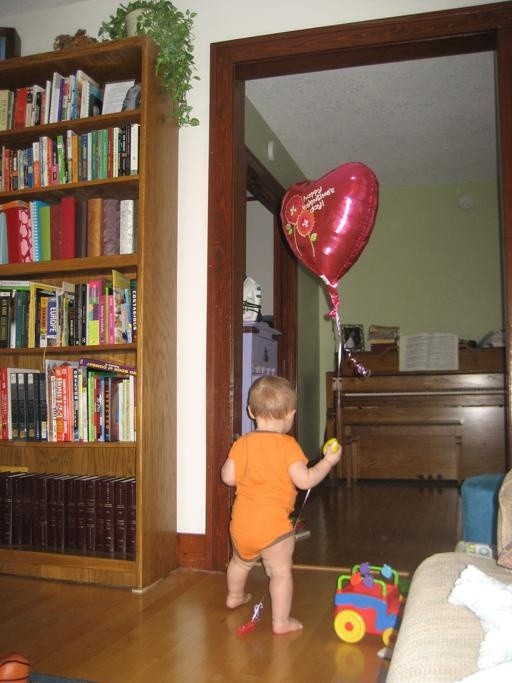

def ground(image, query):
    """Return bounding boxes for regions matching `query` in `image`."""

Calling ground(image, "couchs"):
[385,467,512,683]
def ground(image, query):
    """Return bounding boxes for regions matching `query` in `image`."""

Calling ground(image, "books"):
[367,324,477,372]
[0,470,137,554]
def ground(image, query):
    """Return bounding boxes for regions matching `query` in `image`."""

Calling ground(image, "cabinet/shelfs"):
[0,32,176,590]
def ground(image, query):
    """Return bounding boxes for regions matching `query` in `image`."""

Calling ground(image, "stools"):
[454,475,503,549]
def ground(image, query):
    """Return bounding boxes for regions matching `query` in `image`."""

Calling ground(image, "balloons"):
[279,161,380,307]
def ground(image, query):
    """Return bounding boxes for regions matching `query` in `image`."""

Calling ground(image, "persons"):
[220,374,341,635]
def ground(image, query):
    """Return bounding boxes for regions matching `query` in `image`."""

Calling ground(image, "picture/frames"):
[341,324,366,353]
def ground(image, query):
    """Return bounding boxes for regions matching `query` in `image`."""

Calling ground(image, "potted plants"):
[99,1,204,129]
